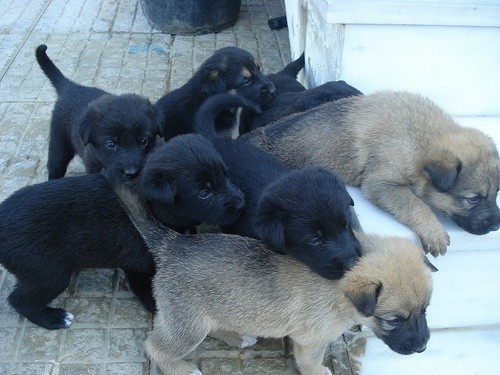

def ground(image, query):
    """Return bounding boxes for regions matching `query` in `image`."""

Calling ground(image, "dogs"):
[241,88,500,259]
[239,52,365,133]
[107,171,440,375]
[36,43,165,186]
[189,93,365,283]
[1,132,247,331]
[154,46,275,142]
